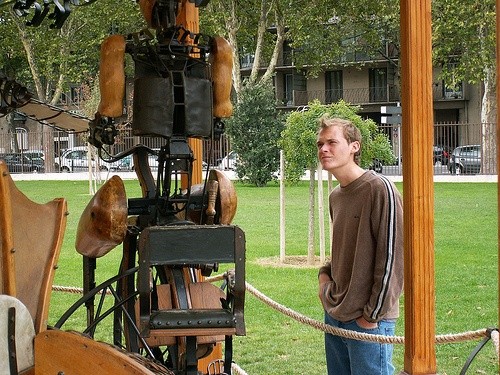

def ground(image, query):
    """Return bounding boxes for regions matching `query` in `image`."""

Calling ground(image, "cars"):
[218,151,242,169]
[0,147,177,174]
[201,161,212,168]
[370,158,382,172]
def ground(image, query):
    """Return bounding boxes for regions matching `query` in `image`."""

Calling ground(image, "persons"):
[317,116,404,375]
[75,0,246,375]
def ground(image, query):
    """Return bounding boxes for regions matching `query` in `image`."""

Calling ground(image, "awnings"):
[0,90,100,195]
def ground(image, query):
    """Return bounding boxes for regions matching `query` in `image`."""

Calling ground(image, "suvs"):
[394,144,453,166]
[447,145,482,175]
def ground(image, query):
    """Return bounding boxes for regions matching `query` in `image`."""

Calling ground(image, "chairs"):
[130,223,247,349]
